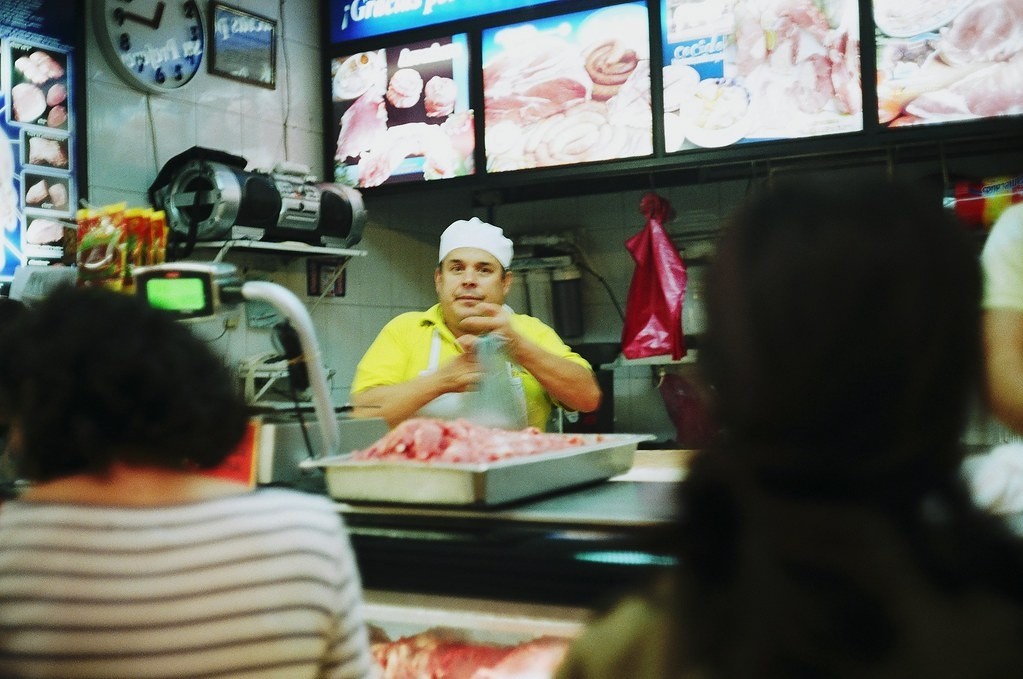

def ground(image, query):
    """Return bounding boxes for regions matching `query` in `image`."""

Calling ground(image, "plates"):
[663,113,685,155]
[679,77,754,149]
[872,1,969,38]
[662,65,701,113]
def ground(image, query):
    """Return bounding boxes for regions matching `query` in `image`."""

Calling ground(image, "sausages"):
[524,43,637,166]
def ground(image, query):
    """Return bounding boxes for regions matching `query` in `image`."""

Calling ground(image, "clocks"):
[89,0,208,96]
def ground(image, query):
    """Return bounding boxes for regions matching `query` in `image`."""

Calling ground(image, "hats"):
[700,175,983,472]
[439,216,515,270]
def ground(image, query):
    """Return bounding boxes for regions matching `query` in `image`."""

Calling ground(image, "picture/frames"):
[208,0,277,91]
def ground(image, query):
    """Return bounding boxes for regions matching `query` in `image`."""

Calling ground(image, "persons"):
[975,201,1023,442]
[551,173,1023,679]
[351,215,602,436]
[0,281,388,679]
